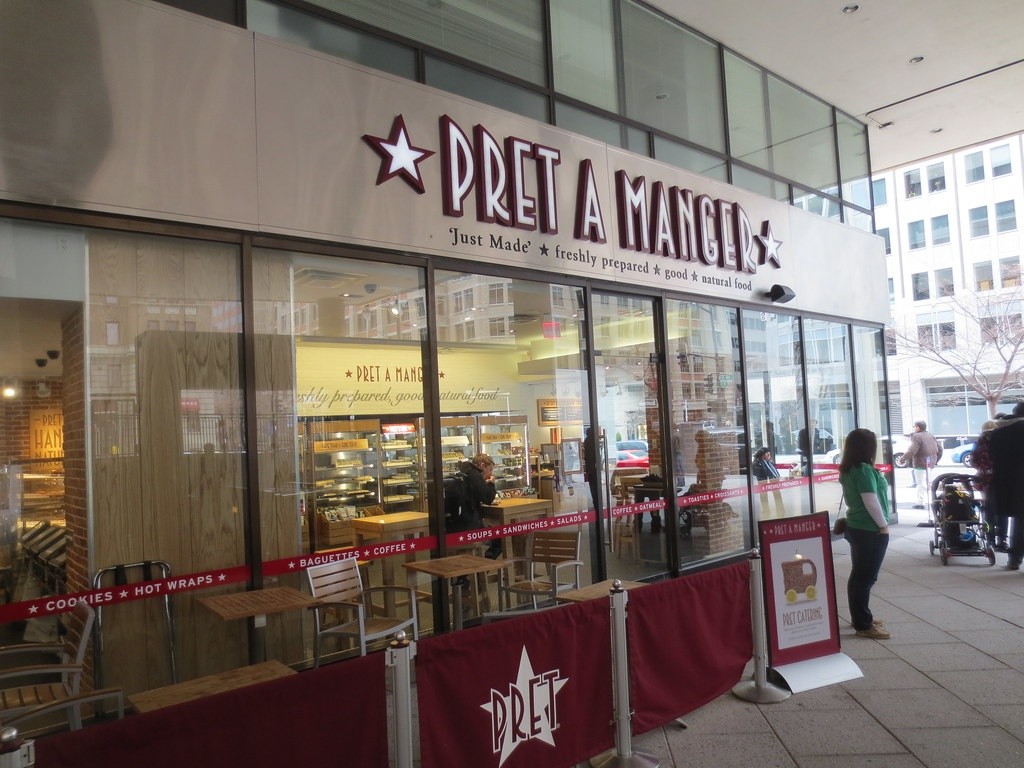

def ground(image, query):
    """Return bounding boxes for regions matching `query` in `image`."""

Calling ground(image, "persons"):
[972,402,1024,570]
[899,421,943,509]
[752,448,785,518]
[767,414,807,454]
[675,431,724,532]
[446,453,504,588]
[839,429,890,639]
[798,419,819,477]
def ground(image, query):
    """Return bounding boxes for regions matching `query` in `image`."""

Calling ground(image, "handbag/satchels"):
[834,517,846,534]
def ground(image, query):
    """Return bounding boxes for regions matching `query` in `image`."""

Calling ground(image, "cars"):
[795,429,833,453]
[616,440,648,451]
[826,433,943,468]
[709,428,746,468]
[616,450,649,467]
[951,444,973,468]
[703,421,715,430]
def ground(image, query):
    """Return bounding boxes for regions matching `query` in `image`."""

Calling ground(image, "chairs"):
[481,602,575,624]
[448,541,485,554]
[0,600,125,732]
[497,526,585,612]
[306,547,419,669]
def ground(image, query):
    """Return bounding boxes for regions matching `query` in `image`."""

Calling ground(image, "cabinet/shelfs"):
[360,423,420,510]
[415,415,531,511]
[298,418,385,555]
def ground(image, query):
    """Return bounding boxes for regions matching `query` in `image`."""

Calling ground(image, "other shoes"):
[913,505,924,509]
[1007,561,1019,570]
[986,540,1010,552]
[851,618,884,627]
[856,625,890,638]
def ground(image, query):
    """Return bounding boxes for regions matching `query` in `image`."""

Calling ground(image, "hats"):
[982,420,998,432]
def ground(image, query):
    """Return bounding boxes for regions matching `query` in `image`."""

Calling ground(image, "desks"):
[554,578,651,603]
[124,659,298,715]
[351,512,430,618]
[614,473,649,560]
[480,498,554,608]
[402,555,513,633]
[198,585,324,666]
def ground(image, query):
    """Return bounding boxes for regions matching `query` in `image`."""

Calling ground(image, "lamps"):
[765,284,795,304]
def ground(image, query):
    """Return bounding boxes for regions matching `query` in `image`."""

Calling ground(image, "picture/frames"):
[561,437,582,475]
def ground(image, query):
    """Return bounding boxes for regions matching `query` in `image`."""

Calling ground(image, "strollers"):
[929,472,996,566]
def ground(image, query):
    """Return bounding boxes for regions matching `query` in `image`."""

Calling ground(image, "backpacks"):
[443,473,470,532]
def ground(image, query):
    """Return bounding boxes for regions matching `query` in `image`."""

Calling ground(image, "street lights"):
[391,293,402,338]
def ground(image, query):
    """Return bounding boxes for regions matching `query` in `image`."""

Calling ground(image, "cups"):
[544,453,549,462]
